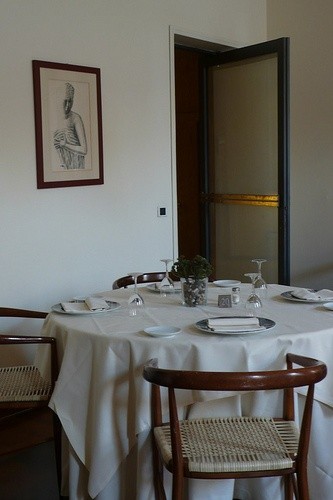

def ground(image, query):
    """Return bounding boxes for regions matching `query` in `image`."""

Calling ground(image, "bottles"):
[232,288,240,304]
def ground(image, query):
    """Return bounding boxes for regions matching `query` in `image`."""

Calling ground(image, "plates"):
[144,326,183,338]
[51,299,120,315]
[280,289,333,302]
[147,283,183,292]
[212,279,241,287]
[195,316,276,334]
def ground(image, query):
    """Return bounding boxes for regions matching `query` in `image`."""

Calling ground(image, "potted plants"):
[173,254,214,305]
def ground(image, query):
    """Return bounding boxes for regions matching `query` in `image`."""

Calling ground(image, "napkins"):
[207,317,265,332]
[61,295,110,313]
[290,289,333,301]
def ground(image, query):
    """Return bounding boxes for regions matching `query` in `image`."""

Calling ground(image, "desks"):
[32,282,333,500]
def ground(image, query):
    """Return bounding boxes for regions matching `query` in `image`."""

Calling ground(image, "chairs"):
[0,306,69,500]
[143,353,328,500]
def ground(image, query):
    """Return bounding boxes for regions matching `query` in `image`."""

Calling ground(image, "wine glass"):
[250,259,268,303]
[158,258,176,300]
[244,273,263,314]
[127,271,146,320]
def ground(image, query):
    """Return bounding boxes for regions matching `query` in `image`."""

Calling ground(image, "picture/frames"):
[31,59,105,189]
